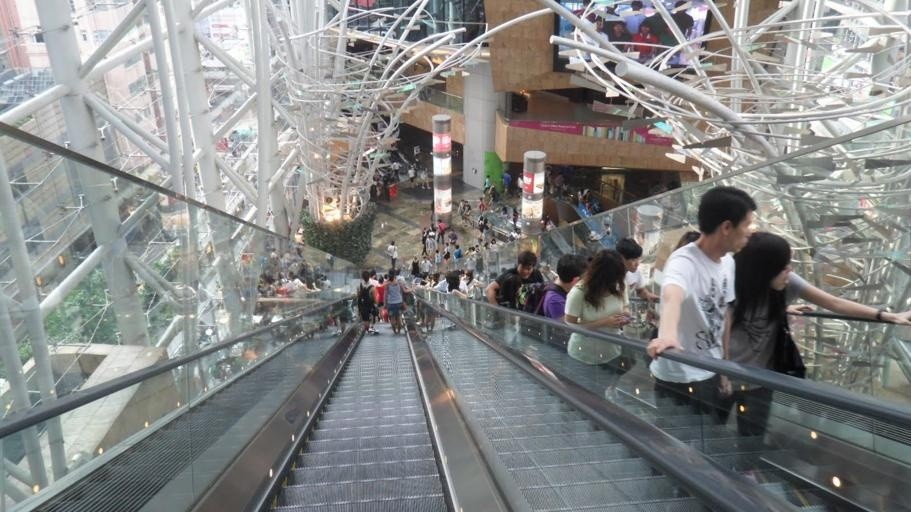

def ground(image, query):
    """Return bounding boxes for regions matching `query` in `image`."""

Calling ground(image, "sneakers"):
[366,327,401,336]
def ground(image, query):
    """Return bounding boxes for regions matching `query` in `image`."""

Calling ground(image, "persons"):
[573,0,694,64]
[271,248,281,262]
[351,144,911,484]
[295,228,304,258]
[255,266,348,341]
[326,254,336,270]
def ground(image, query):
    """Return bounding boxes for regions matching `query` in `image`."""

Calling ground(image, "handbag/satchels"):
[776,325,806,380]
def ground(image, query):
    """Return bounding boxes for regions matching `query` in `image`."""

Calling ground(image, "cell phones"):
[620,314,636,323]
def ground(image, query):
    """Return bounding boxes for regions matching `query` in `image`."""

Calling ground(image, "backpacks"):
[523,281,566,317]
[358,283,375,312]
